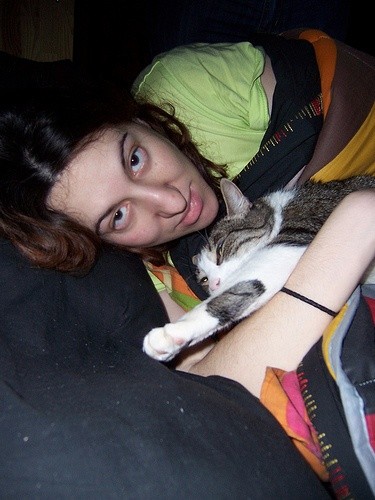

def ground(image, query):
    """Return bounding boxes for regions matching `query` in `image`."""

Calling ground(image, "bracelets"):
[282,286,339,318]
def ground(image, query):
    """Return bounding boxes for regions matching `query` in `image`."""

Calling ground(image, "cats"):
[140,171,375,362]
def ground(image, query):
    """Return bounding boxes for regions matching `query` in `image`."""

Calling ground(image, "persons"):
[0,39,375,399]
